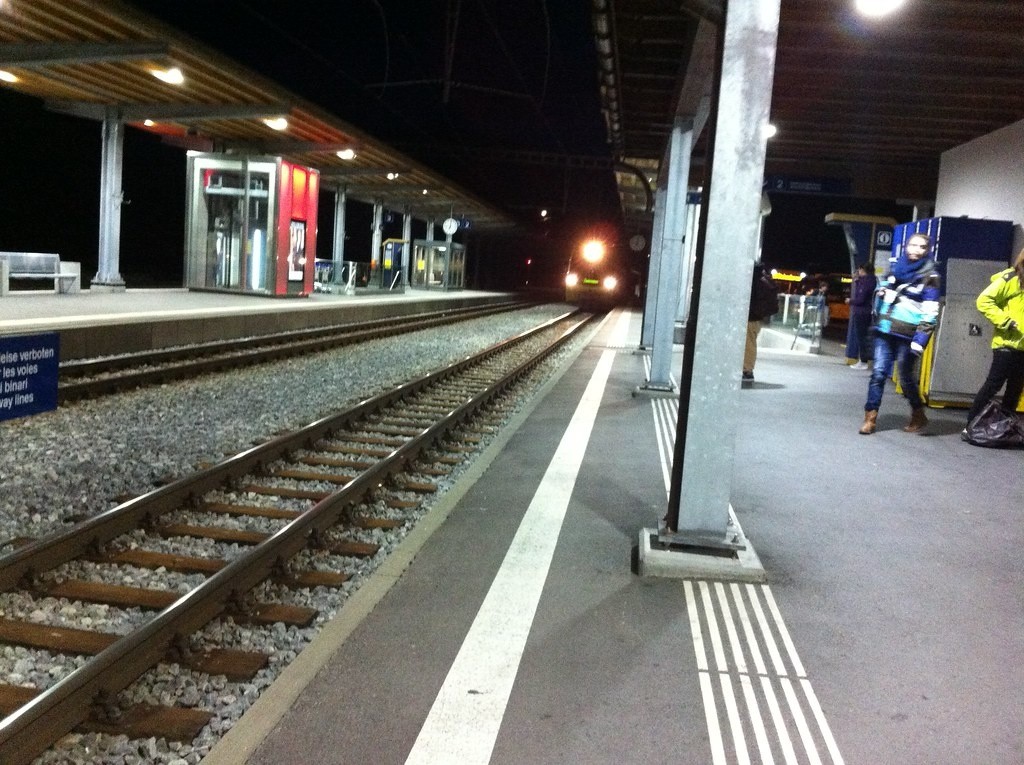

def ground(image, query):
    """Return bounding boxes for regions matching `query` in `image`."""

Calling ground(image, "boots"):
[903,408,929,432]
[860,408,878,434]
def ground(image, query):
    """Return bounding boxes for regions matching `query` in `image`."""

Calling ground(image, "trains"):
[563,221,624,312]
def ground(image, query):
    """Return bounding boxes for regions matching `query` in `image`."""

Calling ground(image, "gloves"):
[1008,320,1018,330]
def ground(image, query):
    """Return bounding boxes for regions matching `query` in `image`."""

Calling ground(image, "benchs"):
[0,252,81,297]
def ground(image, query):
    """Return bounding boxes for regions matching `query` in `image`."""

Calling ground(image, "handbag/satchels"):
[967,398,1024,449]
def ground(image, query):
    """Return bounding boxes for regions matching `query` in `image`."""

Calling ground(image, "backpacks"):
[754,268,779,317]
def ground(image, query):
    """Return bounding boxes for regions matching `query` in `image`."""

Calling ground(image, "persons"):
[810,282,829,305]
[742,263,777,382]
[859,234,941,433]
[845,262,875,370]
[961,248,1024,442]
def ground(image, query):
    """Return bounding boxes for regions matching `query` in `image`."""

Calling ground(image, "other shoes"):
[961,429,967,441]
[849,360,868,370]
[742,372,754,382]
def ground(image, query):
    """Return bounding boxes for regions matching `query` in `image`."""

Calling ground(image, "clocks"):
[443,218,457,235]
[629,235,645,251]
